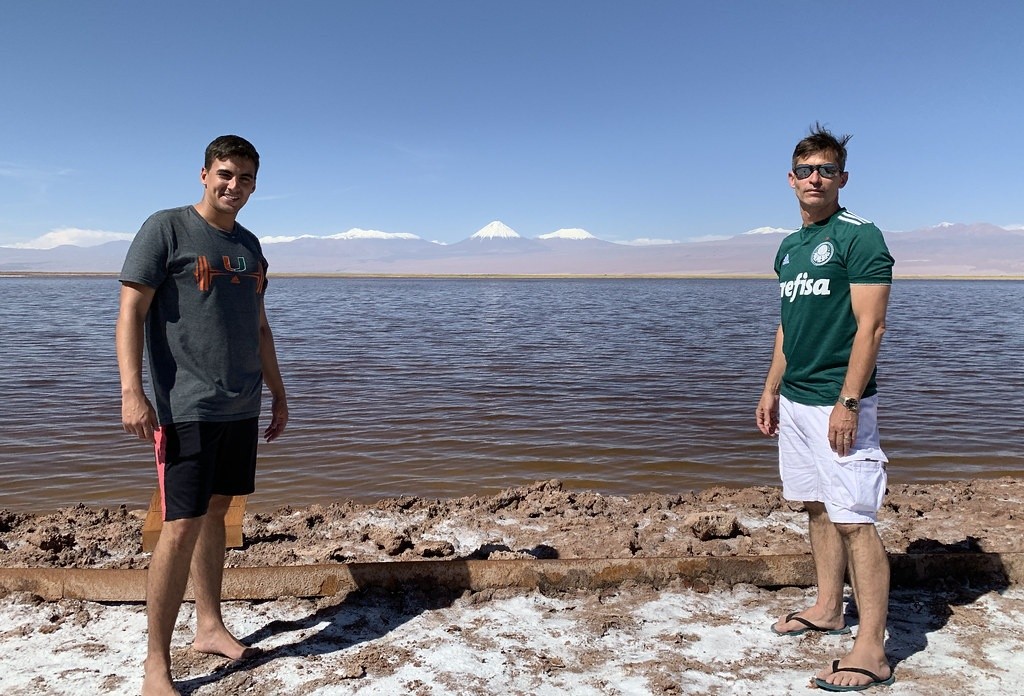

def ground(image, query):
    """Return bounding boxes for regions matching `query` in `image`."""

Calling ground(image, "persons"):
[115,135,289,696]
[757,121,896,691]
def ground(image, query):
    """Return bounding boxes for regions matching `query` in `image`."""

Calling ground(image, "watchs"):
[838,394,860,410]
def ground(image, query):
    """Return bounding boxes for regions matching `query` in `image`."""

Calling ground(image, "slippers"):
[770,612,850,636]
[815,660,895,692]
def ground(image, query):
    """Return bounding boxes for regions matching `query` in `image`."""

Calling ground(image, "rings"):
[843,436,851,439]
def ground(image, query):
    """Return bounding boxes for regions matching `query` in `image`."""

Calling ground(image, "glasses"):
[793,163,843,180]
